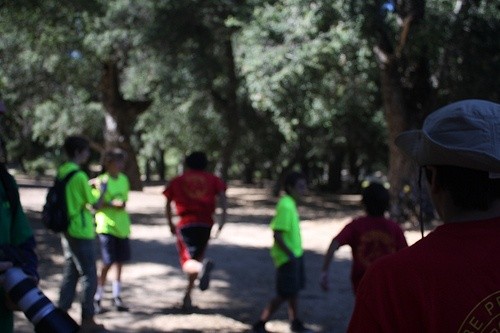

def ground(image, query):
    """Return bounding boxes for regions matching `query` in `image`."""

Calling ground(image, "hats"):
[393,96,500,171]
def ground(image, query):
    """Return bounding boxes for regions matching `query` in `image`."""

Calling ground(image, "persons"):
[0,92,40,333]
[320,181,408,303]
[251,172,316,333]
[162,150,226,313]
[55,136,108,333]
[346,99,500,332]
[87,150,132,314]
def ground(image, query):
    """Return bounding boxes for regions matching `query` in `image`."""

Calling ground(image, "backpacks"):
[42,180,68,232]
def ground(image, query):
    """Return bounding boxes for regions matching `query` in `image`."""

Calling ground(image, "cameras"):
[0,243,81,333]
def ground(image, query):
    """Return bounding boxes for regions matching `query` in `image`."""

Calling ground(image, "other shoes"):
[93,300,102,314]
[111,296,130,311]
[79,318,104,333]
[252,320,269,333]
[182,298,192,314]
[198,259,214,291]
[290,319,314,333]
